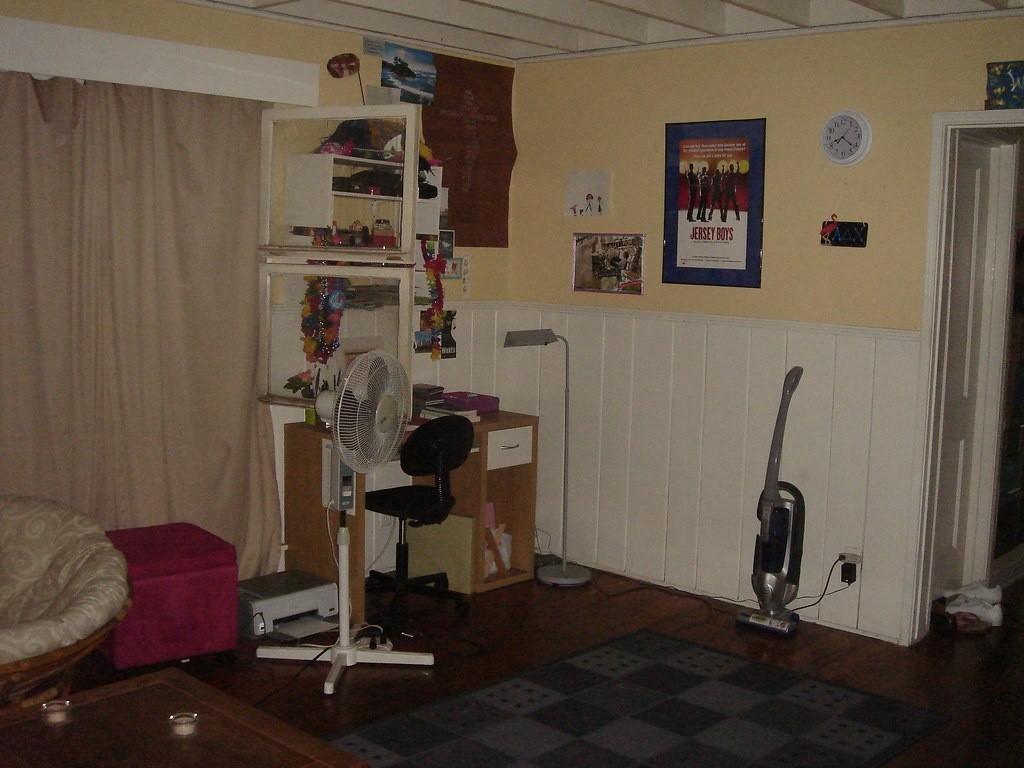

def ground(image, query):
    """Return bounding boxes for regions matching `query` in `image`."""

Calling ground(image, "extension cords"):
[356,637,393,651]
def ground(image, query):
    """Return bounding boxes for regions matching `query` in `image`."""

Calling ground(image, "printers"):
[238,569,339,640]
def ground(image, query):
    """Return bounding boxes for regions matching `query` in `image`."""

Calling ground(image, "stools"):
[91,522,238,670]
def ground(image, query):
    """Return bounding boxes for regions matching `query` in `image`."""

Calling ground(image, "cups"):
[41,700,72,726]
[168,712,198,737]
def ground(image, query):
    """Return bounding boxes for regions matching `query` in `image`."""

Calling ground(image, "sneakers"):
[943,582,1002,604]
[945,597,1003,627]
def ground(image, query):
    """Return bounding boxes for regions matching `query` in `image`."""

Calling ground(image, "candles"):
[41,700,75,725]
[168,711,199,737]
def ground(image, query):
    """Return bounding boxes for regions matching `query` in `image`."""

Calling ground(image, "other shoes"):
[952,611,993,634]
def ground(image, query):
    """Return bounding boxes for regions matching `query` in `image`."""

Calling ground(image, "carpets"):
[315,627,951,768]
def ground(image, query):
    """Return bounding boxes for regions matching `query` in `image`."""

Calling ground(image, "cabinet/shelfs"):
[281,150,442,308]
[487,426,534,471]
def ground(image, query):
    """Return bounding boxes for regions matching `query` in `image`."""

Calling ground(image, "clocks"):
[820,111,868,164]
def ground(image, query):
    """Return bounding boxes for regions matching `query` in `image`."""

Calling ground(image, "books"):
[412,382,480,423]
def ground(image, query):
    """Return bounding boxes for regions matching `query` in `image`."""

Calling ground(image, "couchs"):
[0,493,134,725]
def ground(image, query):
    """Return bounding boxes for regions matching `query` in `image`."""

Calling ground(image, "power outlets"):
[839,552,862,564]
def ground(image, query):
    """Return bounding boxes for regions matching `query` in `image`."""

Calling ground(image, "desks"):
[0,667,373,768]
[284,409,538,593]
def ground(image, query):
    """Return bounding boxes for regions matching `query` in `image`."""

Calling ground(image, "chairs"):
[365,413,474,635]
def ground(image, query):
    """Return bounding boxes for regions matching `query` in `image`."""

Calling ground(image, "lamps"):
[504,329,592,586]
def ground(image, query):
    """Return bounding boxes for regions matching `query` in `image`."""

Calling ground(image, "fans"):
[256,347,434,695]
